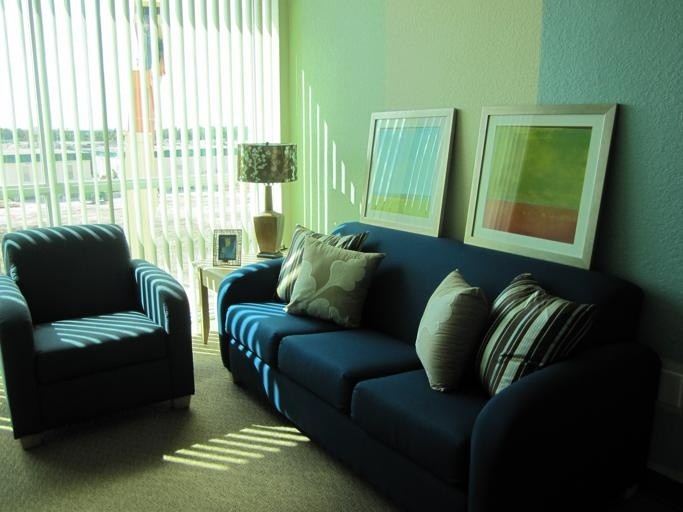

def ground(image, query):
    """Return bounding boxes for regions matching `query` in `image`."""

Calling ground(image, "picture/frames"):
[462,102,621,271]
[358,107,458,239]
[212,228,241,267]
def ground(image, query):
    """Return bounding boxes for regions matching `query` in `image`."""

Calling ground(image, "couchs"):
[216,221,662,512]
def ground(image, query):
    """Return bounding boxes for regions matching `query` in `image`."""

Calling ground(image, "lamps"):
[236,142,298,260]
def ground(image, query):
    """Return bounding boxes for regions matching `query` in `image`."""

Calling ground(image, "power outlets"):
[658,369,683,408]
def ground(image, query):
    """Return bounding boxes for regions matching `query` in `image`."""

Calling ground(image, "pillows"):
[476,273,599,395]
[275,223,369,300]
[288,237,387,330]
[414,268,488,393]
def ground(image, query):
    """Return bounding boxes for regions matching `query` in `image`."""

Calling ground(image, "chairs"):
[0,223,196,450]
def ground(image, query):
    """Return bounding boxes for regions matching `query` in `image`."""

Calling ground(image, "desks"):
[197,260,287,345]
